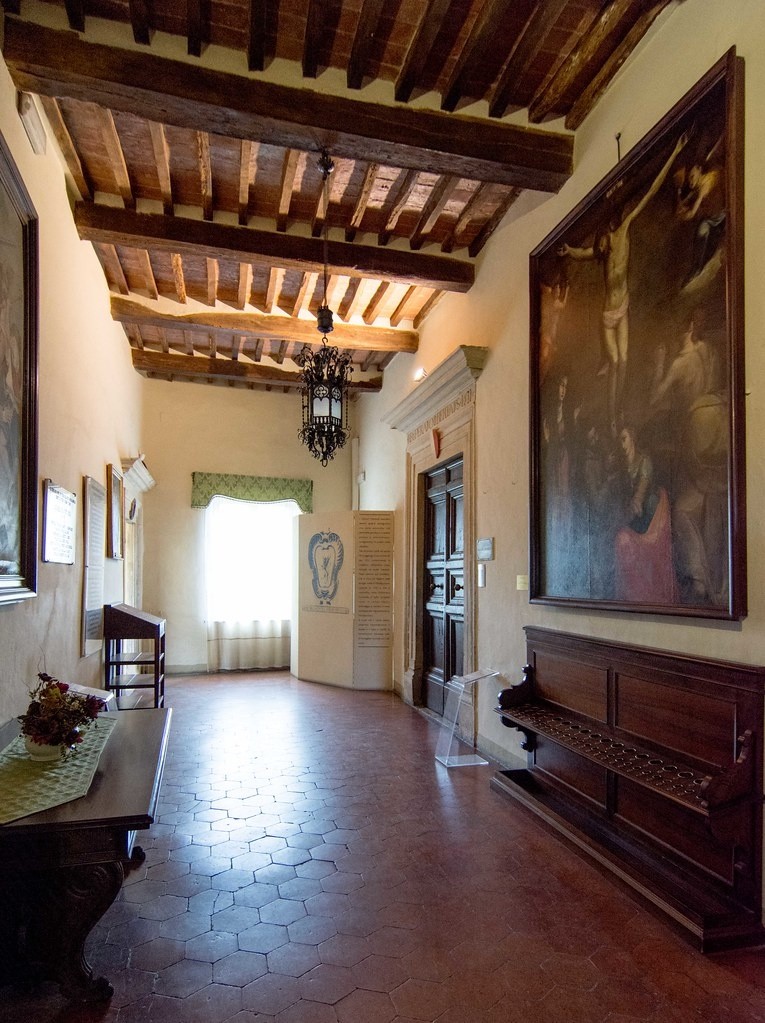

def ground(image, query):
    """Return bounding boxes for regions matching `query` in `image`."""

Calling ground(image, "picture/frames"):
[0,129,42,608]
[527,46,747,622]
[107,463,125,561]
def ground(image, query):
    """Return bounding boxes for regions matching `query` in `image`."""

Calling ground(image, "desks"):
[0,709,173,1007]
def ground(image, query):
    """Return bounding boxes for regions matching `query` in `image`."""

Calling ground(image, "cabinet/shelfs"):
[104,602,165,709]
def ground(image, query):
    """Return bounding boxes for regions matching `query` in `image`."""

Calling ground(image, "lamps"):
[299,151,354,465]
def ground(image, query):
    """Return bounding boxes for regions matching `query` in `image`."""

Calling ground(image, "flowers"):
[20,671,108,745]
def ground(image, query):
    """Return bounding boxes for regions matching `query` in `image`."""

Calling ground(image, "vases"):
[21,730,74,761]
